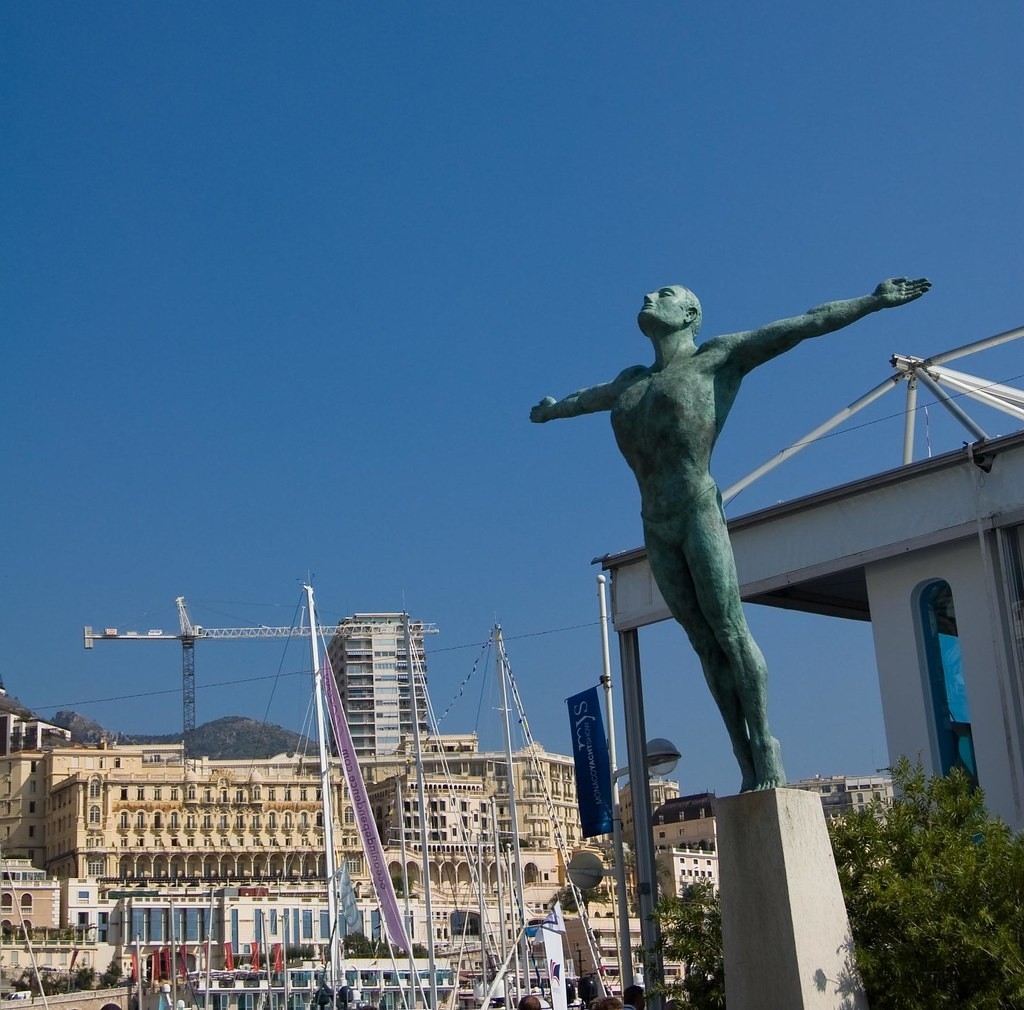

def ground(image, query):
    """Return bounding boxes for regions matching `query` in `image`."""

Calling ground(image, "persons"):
[622,985,646,1010]
[518,995,543,1010]
[531,276,931,792]
[595,996,624,1010]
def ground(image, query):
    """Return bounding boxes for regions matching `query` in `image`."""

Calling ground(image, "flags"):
[535,901,566,943]
[180,945,187,977]
[273,943,283,972]
[339,866,363,933]
[567,688,624,839]
[154,950,161,980]
[164,948,171,980]
[203,942,208,971]
[251,942,259,972]
[224,942,234,970]
[132,952,139,982]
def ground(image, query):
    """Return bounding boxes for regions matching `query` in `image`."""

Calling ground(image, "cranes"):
[84,596,439,758]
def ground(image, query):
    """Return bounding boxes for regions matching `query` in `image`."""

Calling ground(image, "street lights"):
[568,573,682,985]
